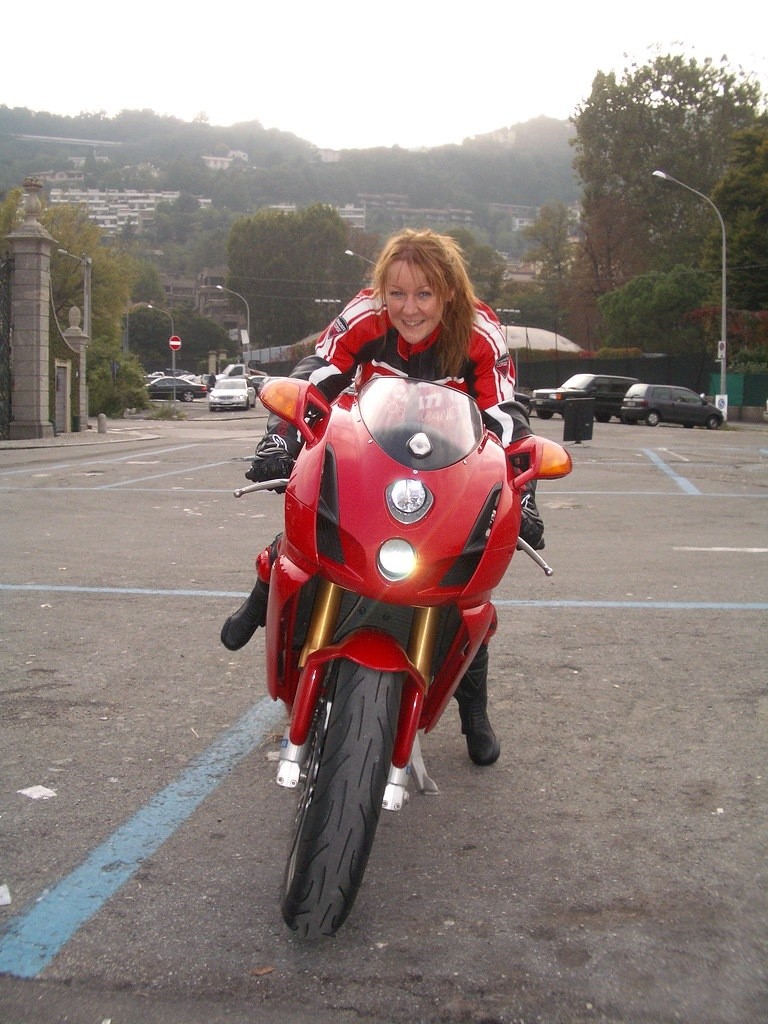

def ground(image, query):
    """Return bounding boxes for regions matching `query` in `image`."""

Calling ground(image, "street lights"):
[216,284,250,365]
[148,303,175,368]
[652,167,727,391]
[345,250,376,266]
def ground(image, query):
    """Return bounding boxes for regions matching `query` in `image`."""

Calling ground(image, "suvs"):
[530,374,640,423]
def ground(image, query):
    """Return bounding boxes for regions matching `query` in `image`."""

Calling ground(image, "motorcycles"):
[234,372,572,939]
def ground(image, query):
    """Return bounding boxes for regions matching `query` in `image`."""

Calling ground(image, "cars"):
[144,364,267,411]
[621,383,724,431]
[514,393,534,421]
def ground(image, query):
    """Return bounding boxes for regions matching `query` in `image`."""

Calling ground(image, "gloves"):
[245,442,294,493]
[516,495,544,552]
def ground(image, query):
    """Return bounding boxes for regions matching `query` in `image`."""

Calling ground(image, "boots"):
[219,576,271,651]
[455,654,501,765]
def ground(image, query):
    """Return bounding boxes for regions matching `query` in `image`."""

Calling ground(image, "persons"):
[209,372,217,392]
[221,229,544,766]
